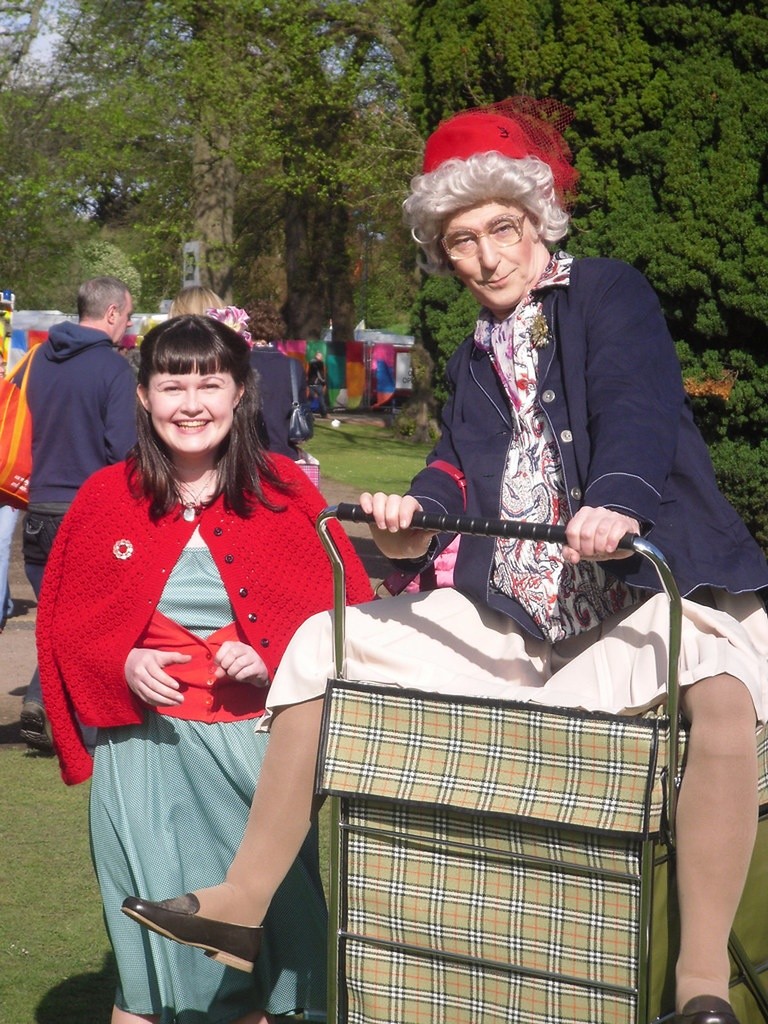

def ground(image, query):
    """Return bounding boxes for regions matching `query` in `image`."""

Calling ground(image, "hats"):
[401,97,581,280]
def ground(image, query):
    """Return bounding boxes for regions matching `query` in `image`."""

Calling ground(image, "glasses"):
[440,210,528,262]
[1,362,7,366]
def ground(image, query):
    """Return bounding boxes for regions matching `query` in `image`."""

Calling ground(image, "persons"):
[119,110,768,1024]
[0,275,382,1024]
[308,352,328,418]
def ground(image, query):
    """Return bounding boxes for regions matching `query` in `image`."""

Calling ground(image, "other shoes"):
[120,893,263,973]
[673,996,740,1024]
[20,701,53,752]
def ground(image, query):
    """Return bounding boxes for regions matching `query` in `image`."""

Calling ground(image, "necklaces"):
[174,465,219,504]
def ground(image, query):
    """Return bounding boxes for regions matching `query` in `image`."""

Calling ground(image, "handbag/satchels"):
[0,343,41,510]
[289,359,315,445]
